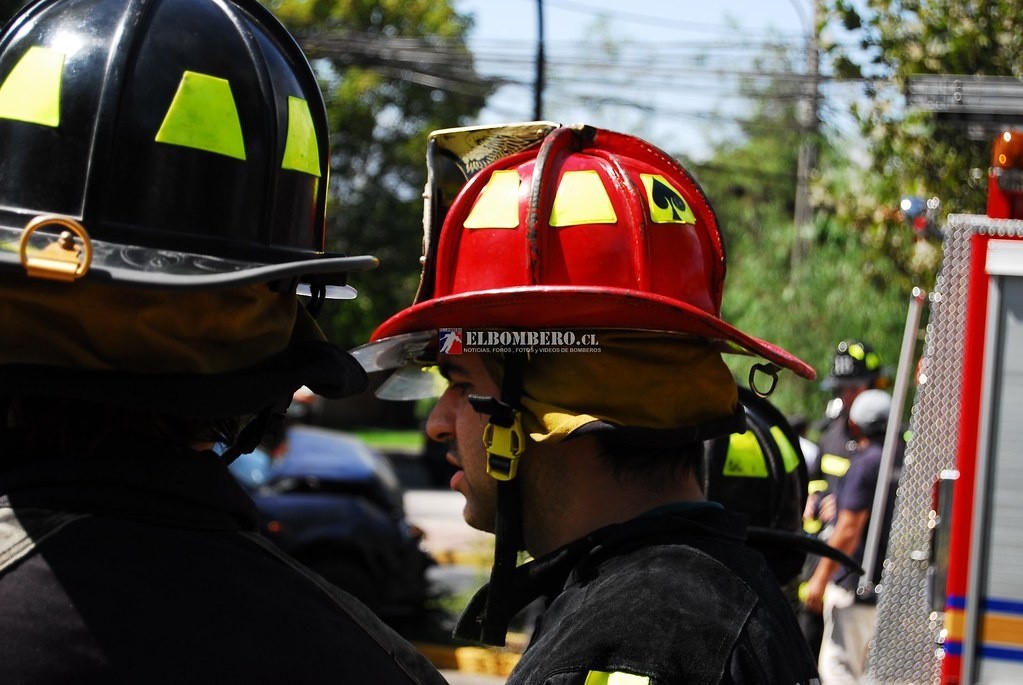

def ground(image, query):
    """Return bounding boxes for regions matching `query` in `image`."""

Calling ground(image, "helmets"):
[694,384,867,577]
[365,125,817,381]
[818,343,887,388]
[0,0,379,288]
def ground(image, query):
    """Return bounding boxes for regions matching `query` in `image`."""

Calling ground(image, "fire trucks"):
[833,121,1022,684]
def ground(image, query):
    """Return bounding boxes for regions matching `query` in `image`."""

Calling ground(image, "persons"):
[798,335,886,649]
[0,0,453,685]
[804,389,901,685]
[347,119,822,685]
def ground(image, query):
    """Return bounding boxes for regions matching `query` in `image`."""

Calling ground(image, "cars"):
[228,425,436,633]
[291,333,453,488]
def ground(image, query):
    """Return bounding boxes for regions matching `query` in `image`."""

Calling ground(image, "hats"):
[850,389,894,434]
